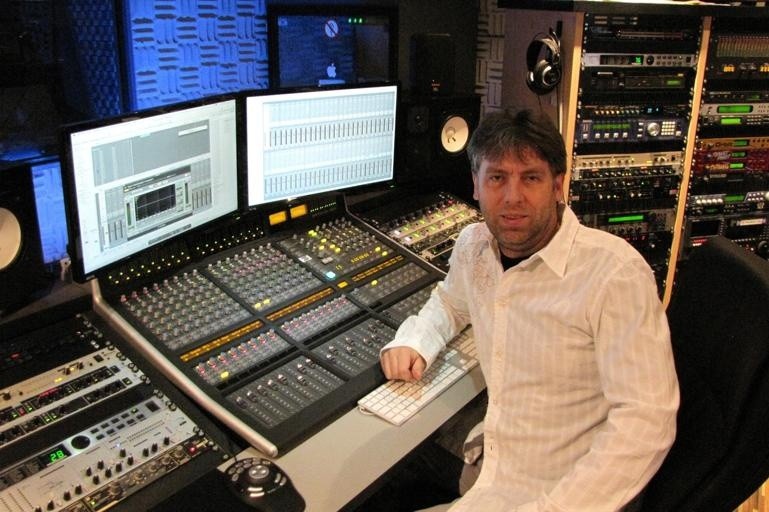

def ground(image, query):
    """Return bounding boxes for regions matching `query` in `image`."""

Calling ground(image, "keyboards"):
[356,327,481,428]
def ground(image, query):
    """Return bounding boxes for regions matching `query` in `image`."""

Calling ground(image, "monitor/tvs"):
[273,9,395,95]
[55,91,242,285]
[242,81,401,217]
[120,0,274,115]
[21,156,68,275]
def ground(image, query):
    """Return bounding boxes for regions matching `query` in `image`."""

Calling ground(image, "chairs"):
[621,236,769,512]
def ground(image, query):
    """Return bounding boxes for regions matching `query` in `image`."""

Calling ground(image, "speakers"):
[403,93,480,199]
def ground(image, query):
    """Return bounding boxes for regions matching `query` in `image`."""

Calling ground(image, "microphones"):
[548,27,558,45]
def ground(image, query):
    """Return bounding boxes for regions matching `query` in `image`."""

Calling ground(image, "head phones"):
[524,33,562,96]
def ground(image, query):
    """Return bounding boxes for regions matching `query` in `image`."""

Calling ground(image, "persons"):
[379,108,680,512]
